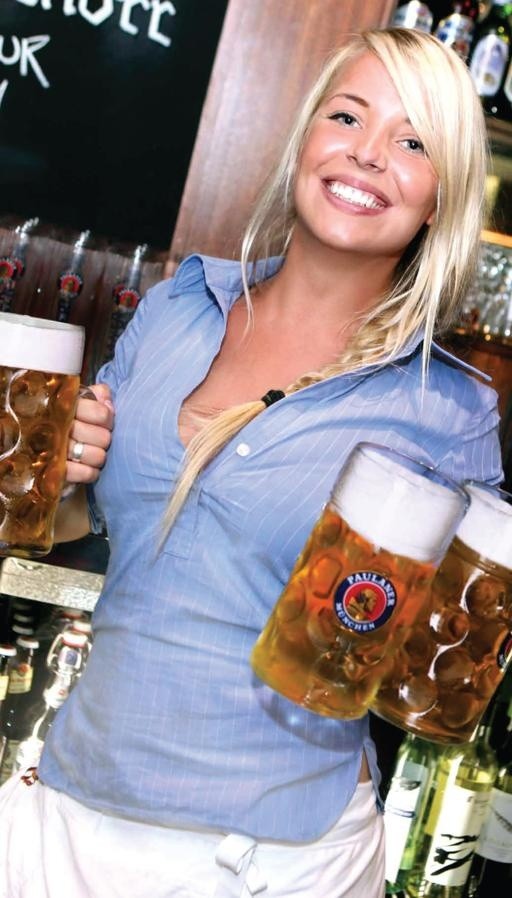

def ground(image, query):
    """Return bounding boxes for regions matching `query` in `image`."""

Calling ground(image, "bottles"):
[0,592,98,788]
[375,708,512,898]
[0,216,148,359]
[391,0,512,127]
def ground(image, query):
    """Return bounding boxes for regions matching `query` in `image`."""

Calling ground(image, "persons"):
[1,27,504,898]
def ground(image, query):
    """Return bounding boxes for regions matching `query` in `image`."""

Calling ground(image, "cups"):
[438,243,512,358]
[249,437,473,726]
[1,311,97,562]
[367,479,512,747]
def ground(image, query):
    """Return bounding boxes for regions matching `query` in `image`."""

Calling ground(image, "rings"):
[70,441,84,463]
